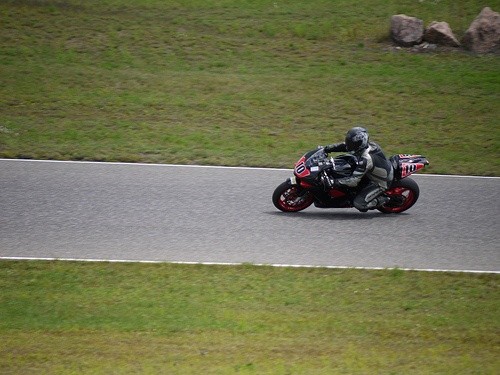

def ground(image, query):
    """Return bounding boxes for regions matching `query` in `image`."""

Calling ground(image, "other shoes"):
[369,196,388,210]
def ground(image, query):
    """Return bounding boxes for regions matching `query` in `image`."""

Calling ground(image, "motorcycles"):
[272,146,430,215]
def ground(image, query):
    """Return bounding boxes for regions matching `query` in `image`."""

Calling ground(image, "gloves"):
[326,176,335,188]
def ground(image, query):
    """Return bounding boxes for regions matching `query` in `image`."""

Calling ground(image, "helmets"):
[344,127,370,153]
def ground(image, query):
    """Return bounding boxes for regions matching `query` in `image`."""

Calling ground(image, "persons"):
[317,127,394,211]
